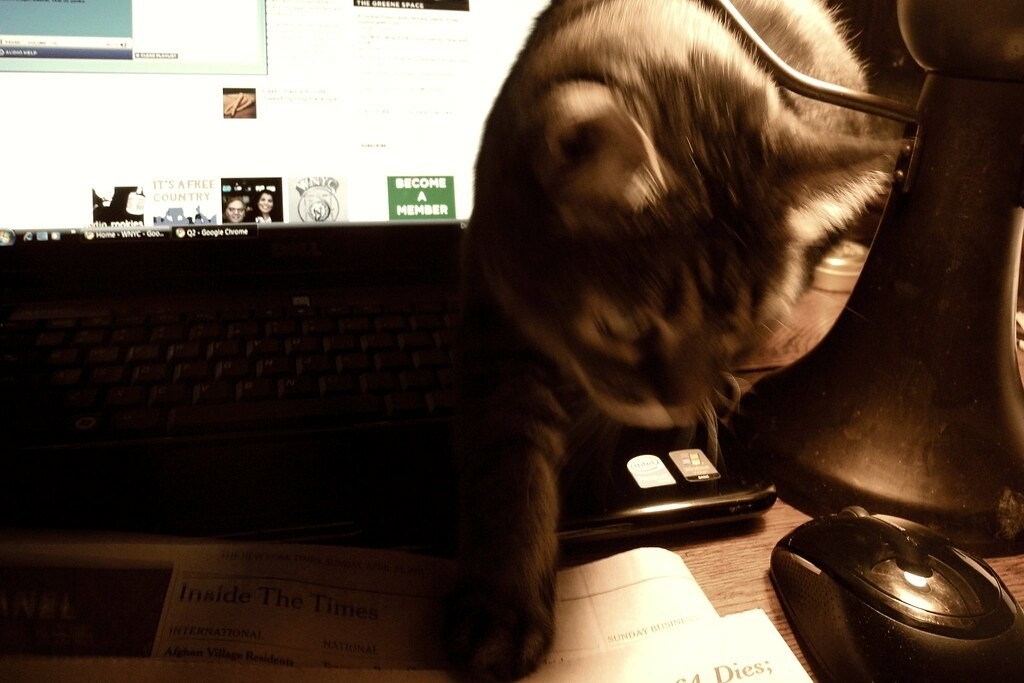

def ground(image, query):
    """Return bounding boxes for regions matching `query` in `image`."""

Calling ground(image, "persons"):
[224,190,279,224]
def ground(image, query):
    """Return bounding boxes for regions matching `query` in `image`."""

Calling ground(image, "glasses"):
[227,208,245,213]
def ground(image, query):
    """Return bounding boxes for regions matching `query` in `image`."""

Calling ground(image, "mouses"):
[770,504,1024,683]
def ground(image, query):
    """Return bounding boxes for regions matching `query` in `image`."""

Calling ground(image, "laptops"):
[0,1,781,552]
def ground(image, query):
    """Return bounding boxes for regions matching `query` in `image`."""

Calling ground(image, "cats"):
[446,0,910,683]
